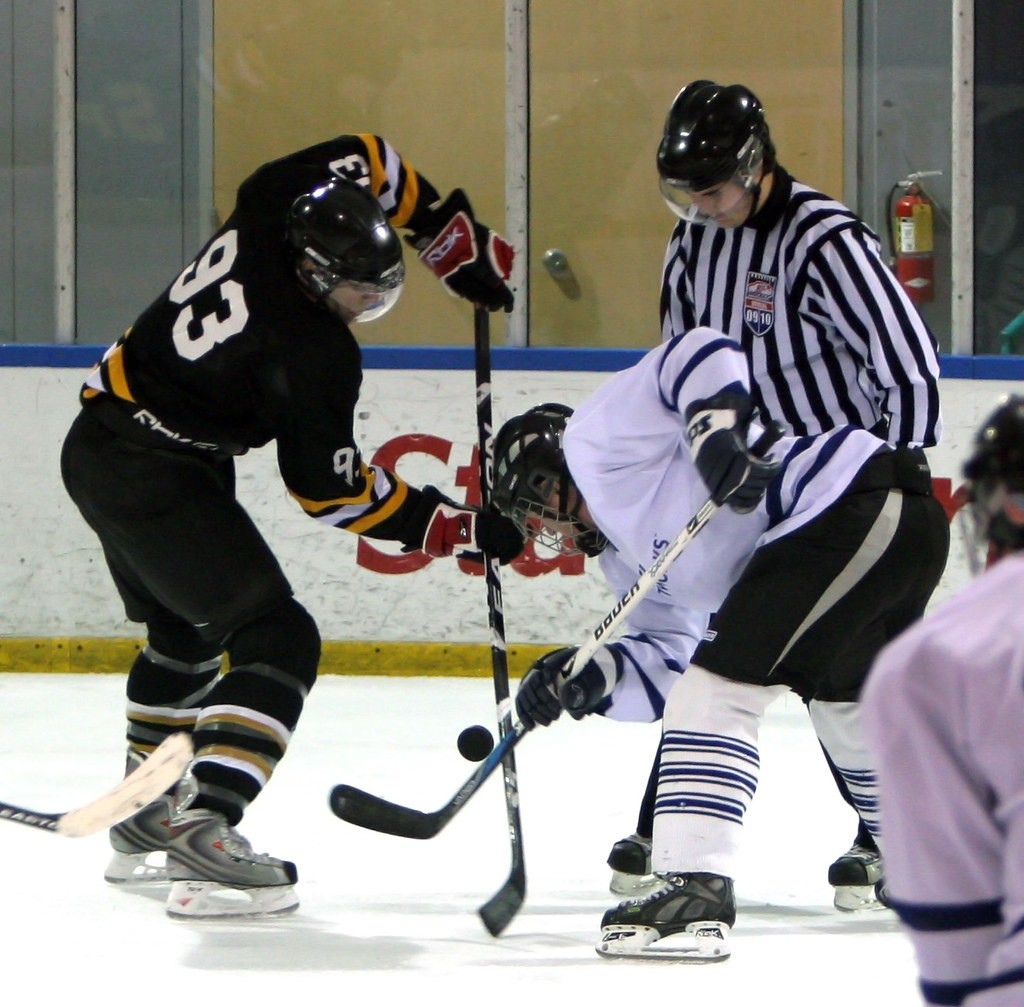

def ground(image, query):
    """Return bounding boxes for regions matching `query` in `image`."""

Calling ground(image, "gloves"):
[399,484,524,577]
[403,188,515,315]
[685,397,783,516]
[513,646,623,732]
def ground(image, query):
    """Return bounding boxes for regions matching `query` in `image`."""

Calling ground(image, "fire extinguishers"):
[894,168,943,303]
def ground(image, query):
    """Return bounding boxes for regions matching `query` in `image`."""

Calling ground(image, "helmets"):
[975,397,1024,494]
[656,79,769,193]
[281,177,406,289]
[491,402,612,558]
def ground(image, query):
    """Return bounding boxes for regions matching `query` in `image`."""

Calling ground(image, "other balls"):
[456,724,494,764]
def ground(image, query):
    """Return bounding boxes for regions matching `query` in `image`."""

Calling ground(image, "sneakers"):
[164,777,298,916]
[105,750,169,881]
[606,833,660,894]
[827,842,889,914]
[596,870,736,960]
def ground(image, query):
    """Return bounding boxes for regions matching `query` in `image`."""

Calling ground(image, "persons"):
[60,134,534,887]
[657,80,941,886]
[494,326,951,937]
[865,394,1024,1007]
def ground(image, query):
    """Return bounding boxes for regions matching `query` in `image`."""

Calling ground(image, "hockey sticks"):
[469,297,530,941]
[326,416,788,845]
[0,729,196,840]
